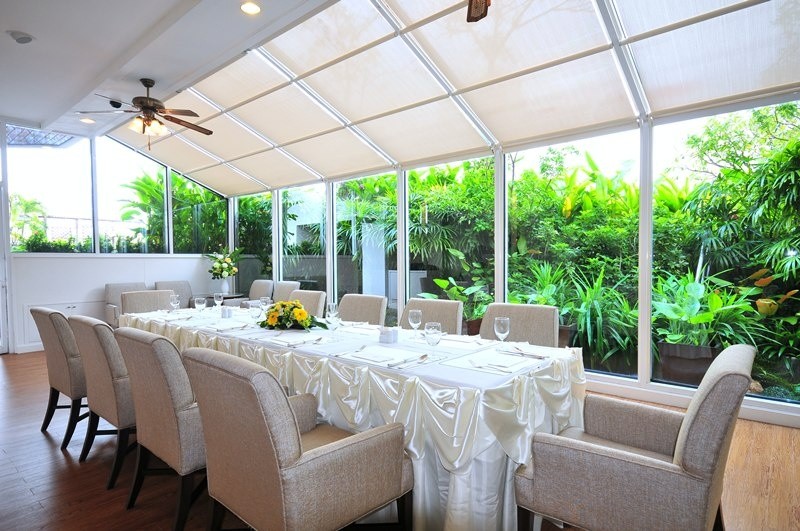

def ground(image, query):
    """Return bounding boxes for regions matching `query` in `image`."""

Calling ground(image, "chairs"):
[30,282,757,531]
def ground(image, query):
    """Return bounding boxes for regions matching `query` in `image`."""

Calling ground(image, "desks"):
[205,291,245,301]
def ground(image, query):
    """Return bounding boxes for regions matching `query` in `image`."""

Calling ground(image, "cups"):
[379,330,398,344]
[221,307,232,318]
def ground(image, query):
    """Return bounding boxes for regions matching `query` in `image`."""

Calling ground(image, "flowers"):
[205,245,245,279]
[260,301,329,332]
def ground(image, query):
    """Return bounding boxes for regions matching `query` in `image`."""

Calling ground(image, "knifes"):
[499,350,545,359]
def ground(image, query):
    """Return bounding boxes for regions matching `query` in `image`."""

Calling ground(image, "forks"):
[468,359,512,373]
[334,344,367,357]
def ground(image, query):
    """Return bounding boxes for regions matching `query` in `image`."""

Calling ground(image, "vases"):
[221,279,228,294]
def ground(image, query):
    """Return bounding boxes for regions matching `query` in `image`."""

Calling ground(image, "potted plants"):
[417,277,519,335]
[652,265,783,386]
[519,260,638,375]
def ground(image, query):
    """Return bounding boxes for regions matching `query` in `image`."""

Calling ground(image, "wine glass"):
[250,297,273,328]
[494,317,510,344]
[214,293,223,310]
[195,298,206,318]
[170,295,180,313]
[425,322,441,356]
[325,303,340,338]
[408,309,421,335]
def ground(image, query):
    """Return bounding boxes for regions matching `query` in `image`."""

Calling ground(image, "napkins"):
[354,344,393,362]
[490,354,526,367]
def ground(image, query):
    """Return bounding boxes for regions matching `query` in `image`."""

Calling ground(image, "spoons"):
[293,337,322,348]
[398,354,428,369]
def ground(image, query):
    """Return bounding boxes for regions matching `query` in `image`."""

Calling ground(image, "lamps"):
[73,77,213,136]
[129,108,168,137]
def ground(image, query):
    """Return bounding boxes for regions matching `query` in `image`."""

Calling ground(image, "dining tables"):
[116,305,584,531]
